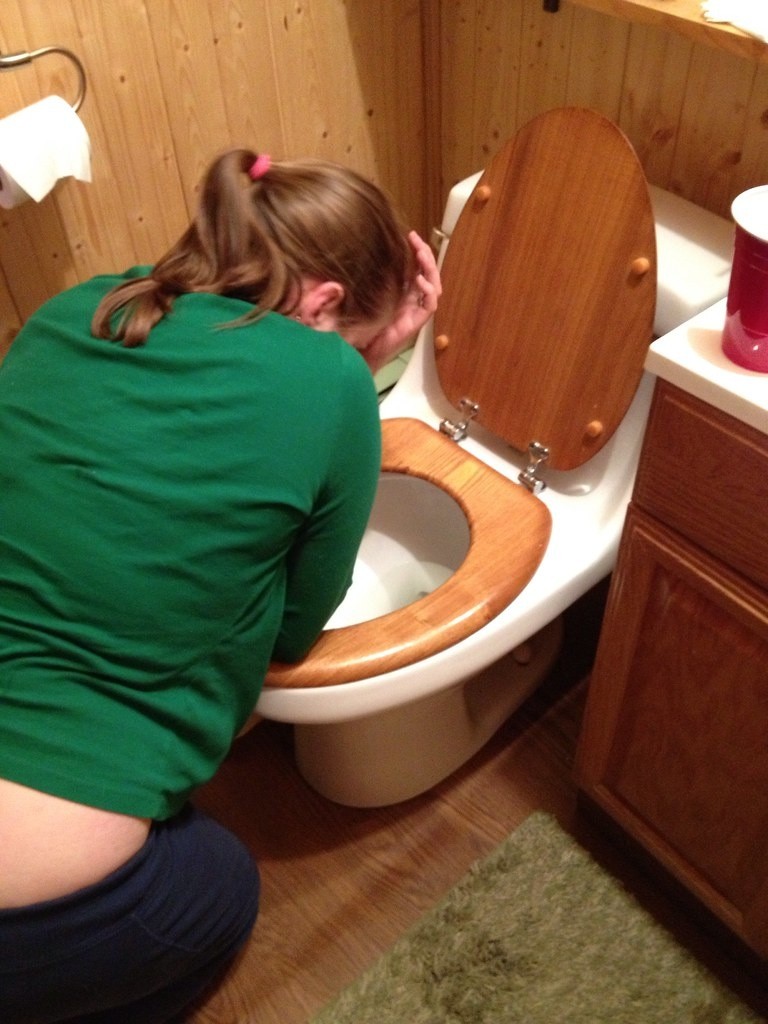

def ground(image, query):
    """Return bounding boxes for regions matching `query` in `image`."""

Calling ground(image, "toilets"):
[208,105,767,813]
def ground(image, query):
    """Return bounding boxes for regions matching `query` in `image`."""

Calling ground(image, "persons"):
[0,150,445,1024]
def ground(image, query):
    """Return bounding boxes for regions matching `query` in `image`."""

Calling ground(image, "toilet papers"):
[0,94,92,210]
